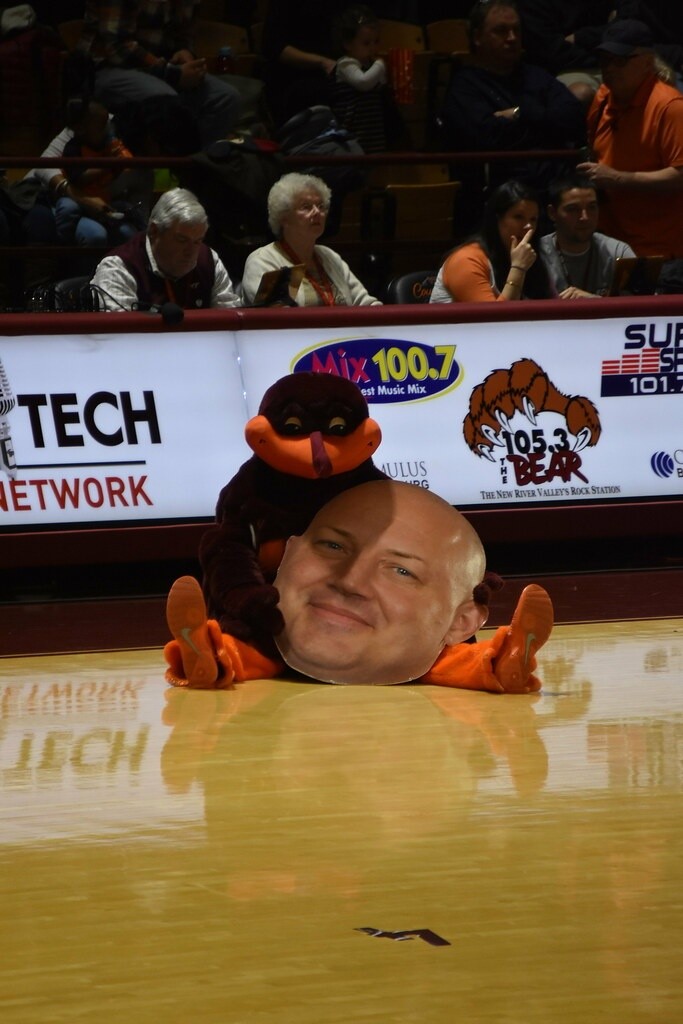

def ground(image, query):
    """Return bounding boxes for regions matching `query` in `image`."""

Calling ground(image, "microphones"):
[130,302,185,323]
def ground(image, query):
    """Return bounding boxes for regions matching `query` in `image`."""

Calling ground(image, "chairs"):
[0,20,471,268]
[386,270,440,304]
[54,275,94,310]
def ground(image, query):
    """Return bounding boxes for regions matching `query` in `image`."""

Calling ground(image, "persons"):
[240,171,383,306]
[272,478,490,685]
[0,0,259,248]
[261,0,412,279]
[539,171,657,299]
[588,19,683,296]
[91,187,241,310]
[435,0,683,248]
[428,182,548,303]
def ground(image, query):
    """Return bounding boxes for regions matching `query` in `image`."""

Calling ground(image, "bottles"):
[576,142,599,190]
[216,47,232,75]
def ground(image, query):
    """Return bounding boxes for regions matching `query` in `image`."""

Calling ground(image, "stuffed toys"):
[164,371,554,694]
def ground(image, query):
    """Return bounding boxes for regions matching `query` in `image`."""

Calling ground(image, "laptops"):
[253,265,307,309]
[610,256,664,296]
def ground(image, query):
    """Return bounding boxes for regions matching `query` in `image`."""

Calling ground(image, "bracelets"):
[511,266,527,272]
[506,281,520,288]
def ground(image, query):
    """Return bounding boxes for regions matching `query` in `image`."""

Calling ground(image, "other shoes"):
[167,576,219,690]
[497,583,554,693]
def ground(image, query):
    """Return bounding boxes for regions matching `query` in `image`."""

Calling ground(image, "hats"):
[596,19,653,55]
